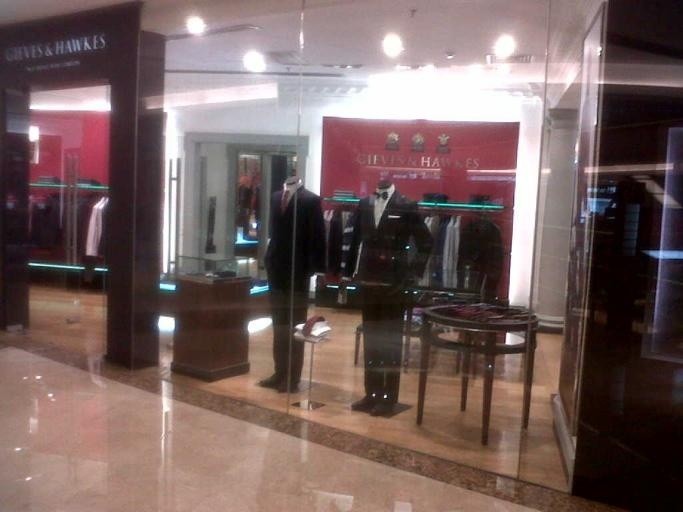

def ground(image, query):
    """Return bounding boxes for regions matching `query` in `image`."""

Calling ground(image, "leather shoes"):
[277,378,300,394]
[350,392,383,413]
[369,396,397,418]
[255,373,287,389]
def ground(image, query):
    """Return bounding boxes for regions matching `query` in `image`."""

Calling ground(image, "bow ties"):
[372,192,390,201]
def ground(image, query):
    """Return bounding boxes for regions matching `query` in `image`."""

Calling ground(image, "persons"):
[259,177,327,393]
[339,180,433,414]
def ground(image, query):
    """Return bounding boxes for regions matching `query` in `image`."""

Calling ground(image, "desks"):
[417,303,536,445]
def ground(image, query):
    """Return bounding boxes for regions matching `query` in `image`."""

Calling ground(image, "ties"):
[279,189,291,213]
[301,315,325,337]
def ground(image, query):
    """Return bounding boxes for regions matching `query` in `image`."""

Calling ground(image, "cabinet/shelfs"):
[170,252,251,379]
[353,271,487,368]
